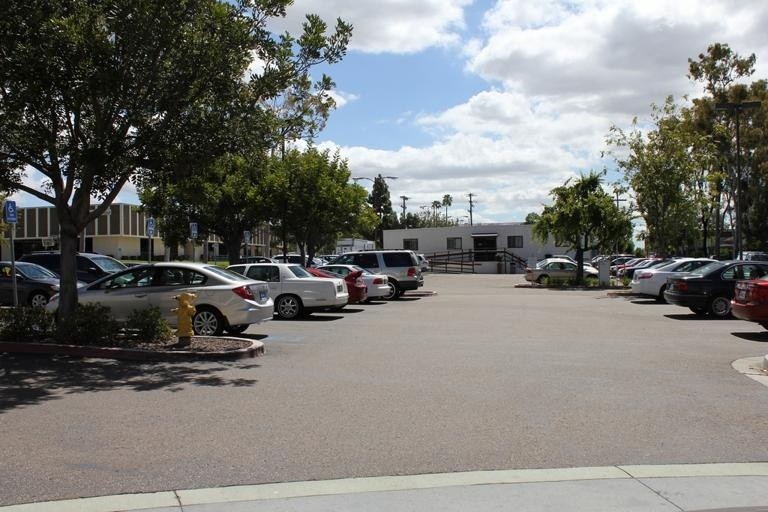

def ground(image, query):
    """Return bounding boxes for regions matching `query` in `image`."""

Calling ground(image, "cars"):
[522,249,768,329]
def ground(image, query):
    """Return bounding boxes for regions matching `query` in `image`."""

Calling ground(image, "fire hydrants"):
[171,292,196,345]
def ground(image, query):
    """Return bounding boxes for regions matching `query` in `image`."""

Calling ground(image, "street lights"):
[351,173,399,192]
[713,99,762,262]
